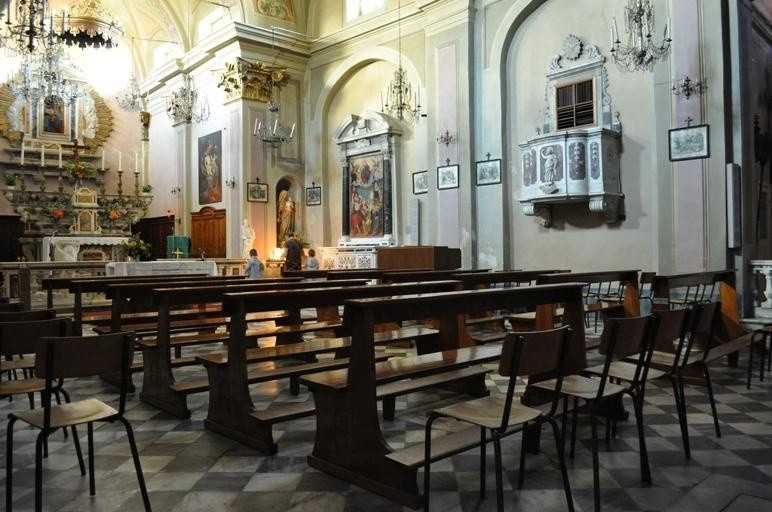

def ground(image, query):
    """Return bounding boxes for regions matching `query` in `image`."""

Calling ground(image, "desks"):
[105,259,217,276]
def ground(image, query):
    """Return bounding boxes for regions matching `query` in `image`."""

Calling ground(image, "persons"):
[350,184,358,213]
[283,232,307,271]
[541,146,558,181]
[277,190,295,246]
[244,249,265,279]
[47,107,61,134]
[351,192,368,235]
[302,250,319,269]
[369,181,384,235]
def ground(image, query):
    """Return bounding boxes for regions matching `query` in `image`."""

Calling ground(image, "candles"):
[59,145,62,170]
[117,149,124,172]
[133,150,140,172]
[21,140,26,165]
[101,147,107,172]
[40,141,48,166]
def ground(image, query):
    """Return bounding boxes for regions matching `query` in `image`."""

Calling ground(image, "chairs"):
[516,314,658,511]
[745,323,771,393]
[623,299,722,462]
[1,305,60,419]
[639,270,656,299]
[581,305,700,488]
[424,323,578,511]
[5,330,153,511]
[0,320,90,475]
[0,298,40,401]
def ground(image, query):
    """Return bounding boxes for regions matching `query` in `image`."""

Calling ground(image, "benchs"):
[67,274,262,350]
[193,278,487,458]
[43,273,219,344]
[132,279,408,424]
[452,267,573,344]
[654,269,738,365]
[281,267,390,280]
[91,275,320,395]
[538,269,640,351]
[387,269,495,278]
[296,281,591,510]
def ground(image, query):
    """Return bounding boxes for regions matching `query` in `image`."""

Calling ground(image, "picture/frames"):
[305,186,322,206]
[436,164,460,190]
[246,182,269,203]
[412,170,429,195]
[476,159,501,186]
[668,124,711,162]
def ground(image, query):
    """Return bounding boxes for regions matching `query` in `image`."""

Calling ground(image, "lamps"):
[671,74,706,101]
[250,25,298,149]
[437,129,457,148]
[0,1,52,51]
[38,1,126,51]
[165,0,211,128]
[5,2,94,117]
[608,0,675,72]
[115,37,144,114]
[378,2,420,127]
